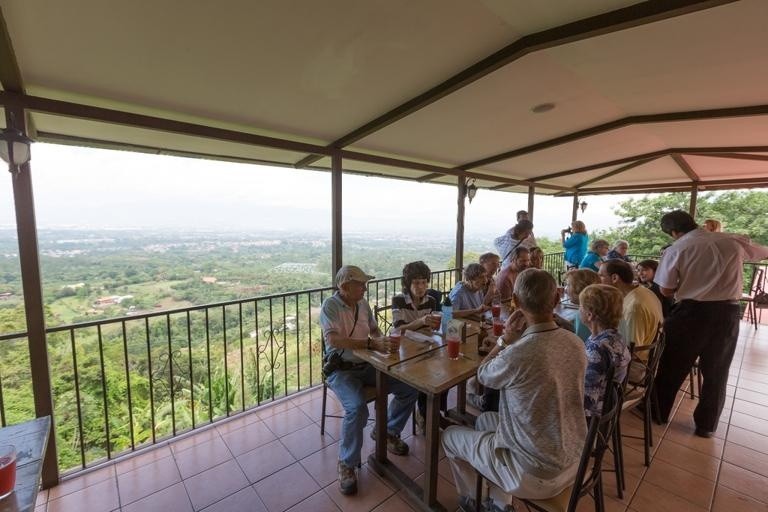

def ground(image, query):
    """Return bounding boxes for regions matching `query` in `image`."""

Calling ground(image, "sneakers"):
[415,409,426,436]
[457,498,516,511]
[694,427,716,438]
[370,425,409,456]
[336,459,357,495]
[636,397,668,425]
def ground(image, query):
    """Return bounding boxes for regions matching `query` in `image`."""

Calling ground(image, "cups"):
[447,333,461,361]
[492,317,506,338]
[491,299,501,317]
[389,328,402,350]
[431,311,442,333]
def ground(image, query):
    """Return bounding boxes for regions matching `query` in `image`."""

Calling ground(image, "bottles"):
[478,313,490,357]
[442,296,454,336]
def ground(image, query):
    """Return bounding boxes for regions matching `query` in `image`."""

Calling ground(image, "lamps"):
[0,109,31,174]
[464,177,477,203]
[576,201,587,213]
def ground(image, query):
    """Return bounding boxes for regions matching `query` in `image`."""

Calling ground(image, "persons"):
[578,283,629,432]
[495,211,537,265]
[562,220,588,271]
[704,219,720,232]
[601,258,671,384]
[391,261,449,434]
[636,210,767,438]
[581,239,634,272]
[442,268,591,511]
[321,264,419,496]
[453,252,501,394]
[465,268,600,411]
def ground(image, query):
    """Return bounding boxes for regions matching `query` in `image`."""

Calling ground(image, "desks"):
[352,315,504,511]
[1,415,52,512]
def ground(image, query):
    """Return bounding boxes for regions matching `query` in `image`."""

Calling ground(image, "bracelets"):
[367,336,373,348]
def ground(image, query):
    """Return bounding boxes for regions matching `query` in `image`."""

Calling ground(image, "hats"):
[336,266,375,287]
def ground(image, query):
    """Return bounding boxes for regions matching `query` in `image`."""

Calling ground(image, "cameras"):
[322,352,343,375]
[566,227,572,232]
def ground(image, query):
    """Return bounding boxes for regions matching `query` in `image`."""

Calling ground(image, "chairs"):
[587,365,625,501]
[739,266,764,330]
[373,304,417,436]
[474,380,622,511]
[435,269,702,447]
[321,329,391,436]
[612,331,665,490]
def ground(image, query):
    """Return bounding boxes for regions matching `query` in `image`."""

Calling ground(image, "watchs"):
[496,334,508,349]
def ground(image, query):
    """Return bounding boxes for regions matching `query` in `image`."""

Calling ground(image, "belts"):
[719,299,739,306]
